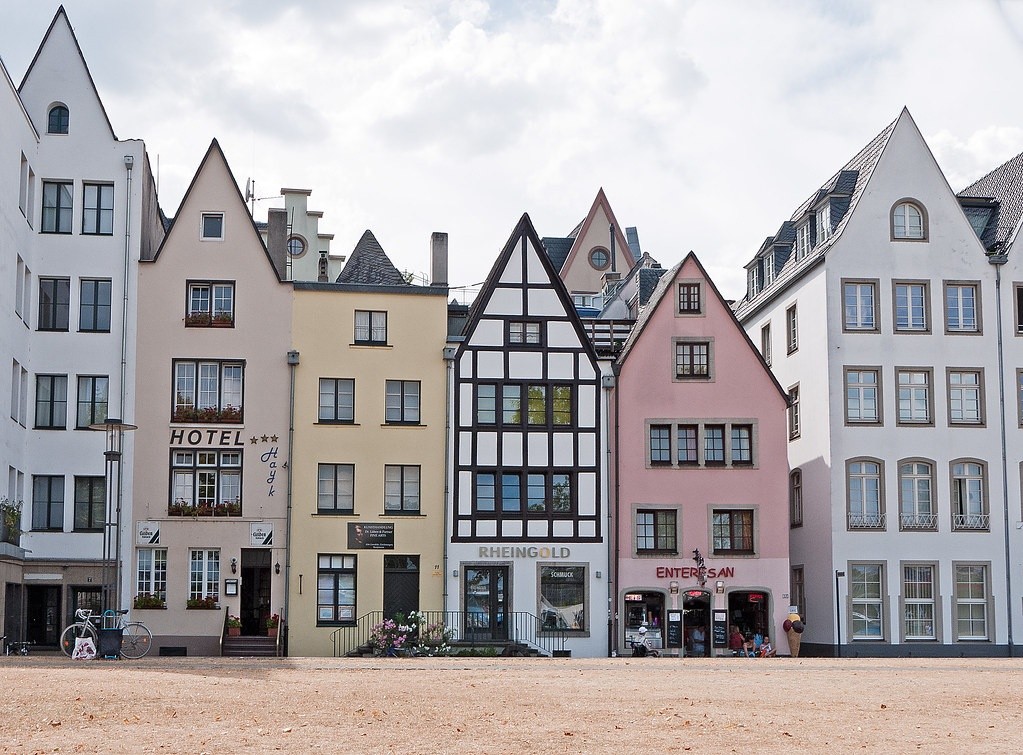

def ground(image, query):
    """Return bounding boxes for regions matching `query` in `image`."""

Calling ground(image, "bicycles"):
[59,608,154,660]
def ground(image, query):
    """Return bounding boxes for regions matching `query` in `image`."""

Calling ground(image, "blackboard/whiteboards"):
[667,609,683,649]
[712,609,728,648]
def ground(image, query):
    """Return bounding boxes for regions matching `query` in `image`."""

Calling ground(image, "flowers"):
[134,594,164,606]
[168,500,242,509]
[228,614,243,625]
[181,307,231,321]
[174,399,243,417]
[264,613,287,626]
[188,594,220,603]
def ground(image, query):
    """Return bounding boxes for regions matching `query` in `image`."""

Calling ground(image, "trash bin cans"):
[99,628,123,661]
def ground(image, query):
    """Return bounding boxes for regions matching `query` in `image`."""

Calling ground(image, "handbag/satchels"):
[71,636,96,660]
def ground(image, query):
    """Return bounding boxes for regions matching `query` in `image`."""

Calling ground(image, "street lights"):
[86,417,140,627]
[833,569,847,658]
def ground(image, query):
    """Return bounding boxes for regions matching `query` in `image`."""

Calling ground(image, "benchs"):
[729,647,762,658]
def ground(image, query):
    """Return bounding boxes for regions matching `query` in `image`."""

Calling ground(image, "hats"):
[747,635,754,639]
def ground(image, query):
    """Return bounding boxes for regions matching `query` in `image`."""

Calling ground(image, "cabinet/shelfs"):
[626,625,663,649]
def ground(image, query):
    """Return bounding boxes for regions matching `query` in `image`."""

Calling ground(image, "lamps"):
[670,581,679,594]
[230,561,237,575]
[715,580,725,594]
[275,562,281,575]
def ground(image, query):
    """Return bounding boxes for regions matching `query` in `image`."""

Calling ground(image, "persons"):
[729,625,777,658]
[691,625,706,655]
[634,627,663,658]
[350,525,367,548]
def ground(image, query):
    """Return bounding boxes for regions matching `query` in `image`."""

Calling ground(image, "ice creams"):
[783,614,804,657]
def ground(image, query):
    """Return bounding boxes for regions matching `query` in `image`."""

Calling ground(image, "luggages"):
[97,610,124,660]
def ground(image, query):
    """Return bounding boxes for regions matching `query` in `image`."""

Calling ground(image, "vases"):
[174,418,242,424]
[168,511,242,516]
[212,320,231,327]
[188,604,216,609]
[228,627,241,636]
[268,627,278,638]
[186,321,209,326]
